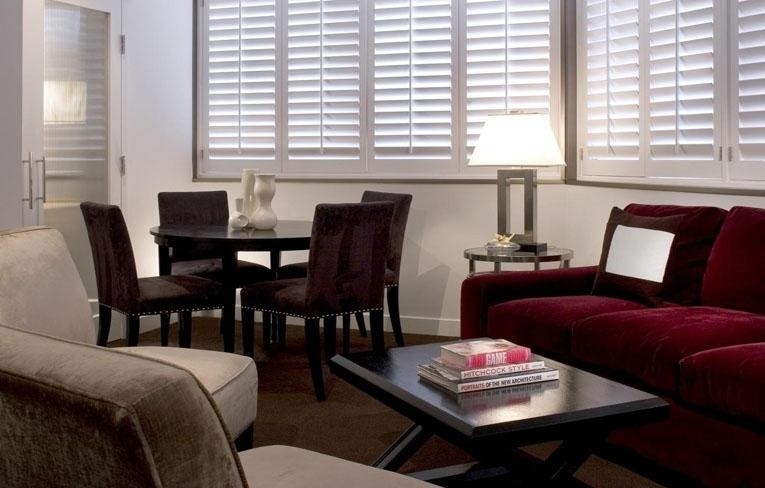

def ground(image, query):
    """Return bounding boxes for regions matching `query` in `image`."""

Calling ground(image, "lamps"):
[466,113,567,255]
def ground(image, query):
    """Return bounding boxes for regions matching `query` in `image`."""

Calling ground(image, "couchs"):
[1,224,449,488]
[461,204,765,488]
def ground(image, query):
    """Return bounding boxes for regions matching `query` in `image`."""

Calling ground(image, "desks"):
[329,337,670,488]
[150,220,313,354]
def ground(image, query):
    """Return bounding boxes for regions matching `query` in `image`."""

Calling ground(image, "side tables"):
[464,247,573,272]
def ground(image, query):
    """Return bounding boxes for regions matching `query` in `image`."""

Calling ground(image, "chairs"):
[158,191,275,350]
[80,201,234,353]
[281,190,412,350]
[241,202,394,402]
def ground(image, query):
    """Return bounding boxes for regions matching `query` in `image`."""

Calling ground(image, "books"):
[416,338,559,393]
